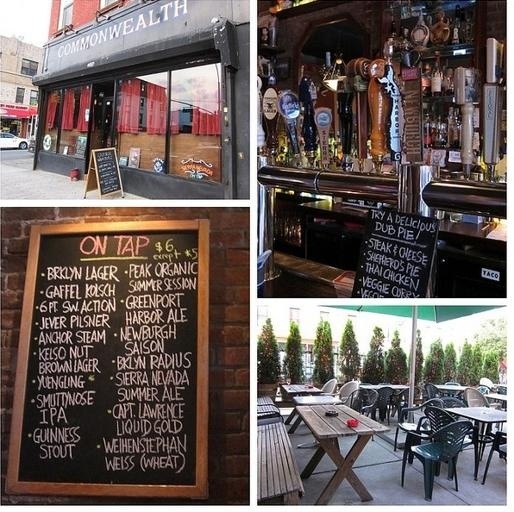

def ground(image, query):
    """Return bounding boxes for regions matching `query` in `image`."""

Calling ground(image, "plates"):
[43,133,52,152]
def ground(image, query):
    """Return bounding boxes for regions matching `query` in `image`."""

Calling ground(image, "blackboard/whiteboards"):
[93,148,122,197]
[350,208,441,298]
[5,218,210,500]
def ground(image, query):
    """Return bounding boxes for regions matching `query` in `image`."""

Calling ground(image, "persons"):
[498,358,507,384]
[28,134,35,152]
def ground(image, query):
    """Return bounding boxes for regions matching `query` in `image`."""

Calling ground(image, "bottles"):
[423,48,462,150]
[273,211,302,244]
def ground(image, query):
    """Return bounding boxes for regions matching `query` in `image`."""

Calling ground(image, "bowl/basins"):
[347,419,359,428]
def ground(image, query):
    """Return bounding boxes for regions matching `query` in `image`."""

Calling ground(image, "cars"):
[28,136,34,153]
[0,133,28,150]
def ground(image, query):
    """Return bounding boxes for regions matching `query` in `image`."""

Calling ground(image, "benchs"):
[258,411,304,505]
[257,393,281,423]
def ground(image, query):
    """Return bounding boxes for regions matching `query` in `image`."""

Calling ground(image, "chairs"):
[323,378,506,502]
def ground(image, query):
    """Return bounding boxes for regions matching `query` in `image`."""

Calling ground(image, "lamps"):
[139,0,147,4]
[60,24,78,39]
[93,11,110,27]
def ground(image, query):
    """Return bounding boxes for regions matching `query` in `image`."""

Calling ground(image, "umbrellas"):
[320,305,506,423]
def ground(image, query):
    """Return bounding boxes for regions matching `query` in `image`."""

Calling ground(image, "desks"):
[282,384,324,401]
[297,405,391,504]
[283,396,344,434]
